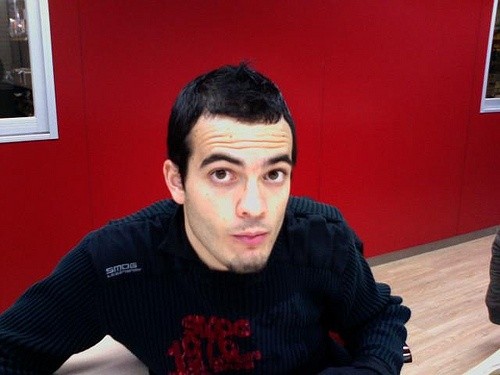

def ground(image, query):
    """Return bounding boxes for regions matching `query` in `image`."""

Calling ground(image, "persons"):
[1,64,411,375]
